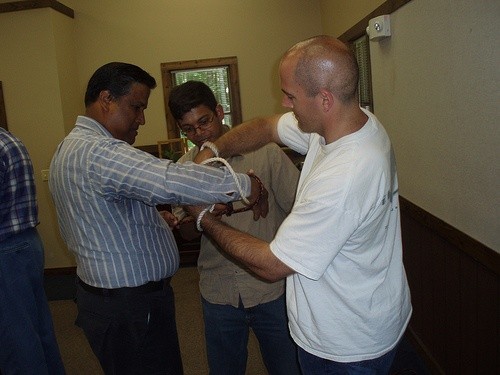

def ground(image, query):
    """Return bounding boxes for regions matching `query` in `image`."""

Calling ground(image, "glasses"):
[176,110,214,138]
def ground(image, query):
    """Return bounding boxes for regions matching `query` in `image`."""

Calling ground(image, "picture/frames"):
[157,138,188,163]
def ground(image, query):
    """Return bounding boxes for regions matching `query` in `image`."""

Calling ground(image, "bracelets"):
[250,172,265,204]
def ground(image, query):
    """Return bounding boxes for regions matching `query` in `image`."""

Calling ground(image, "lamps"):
[366,15,391,40]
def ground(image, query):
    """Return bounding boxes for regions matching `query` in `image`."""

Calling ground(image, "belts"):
[75,275,172,296]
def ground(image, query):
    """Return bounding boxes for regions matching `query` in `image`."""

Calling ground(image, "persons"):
[167,80,303,375]
[0,127,68,375]
[180,34,413,375]
[48,61,270,375]
